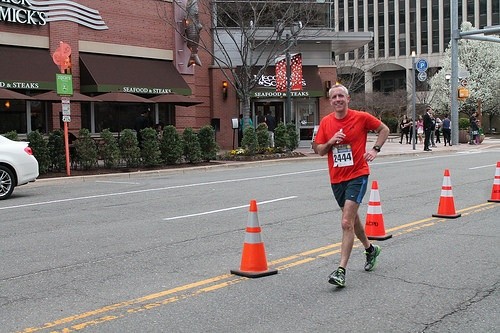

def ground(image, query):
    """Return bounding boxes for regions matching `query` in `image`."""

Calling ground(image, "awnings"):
[0,43,61,90]
[236,66,324,97]
[79,51,192,96]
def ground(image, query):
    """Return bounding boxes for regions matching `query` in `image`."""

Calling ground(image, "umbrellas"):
[0,87,205,135]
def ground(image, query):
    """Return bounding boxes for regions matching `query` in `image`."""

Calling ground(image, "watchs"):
[373,146,380,152]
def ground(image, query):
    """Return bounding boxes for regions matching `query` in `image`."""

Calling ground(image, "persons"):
[459,111,484,145]
[153,125,163,138]
[240,111,278,147]
[134,110,149,149]
[399,107,452,151]
[31,113,43,133]
[314,84,390,285]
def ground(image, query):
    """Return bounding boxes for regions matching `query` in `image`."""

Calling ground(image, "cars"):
[0,135,40,199]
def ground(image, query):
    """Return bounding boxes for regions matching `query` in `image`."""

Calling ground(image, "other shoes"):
[424,147,432,151]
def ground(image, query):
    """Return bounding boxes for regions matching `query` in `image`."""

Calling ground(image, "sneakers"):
[328,268,346,287]
[363,244,381,271]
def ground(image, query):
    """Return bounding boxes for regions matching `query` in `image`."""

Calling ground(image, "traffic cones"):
[430,170,462,219]
[362,179,398,240]
[231,199,281,279]
[486,161,500,202]
